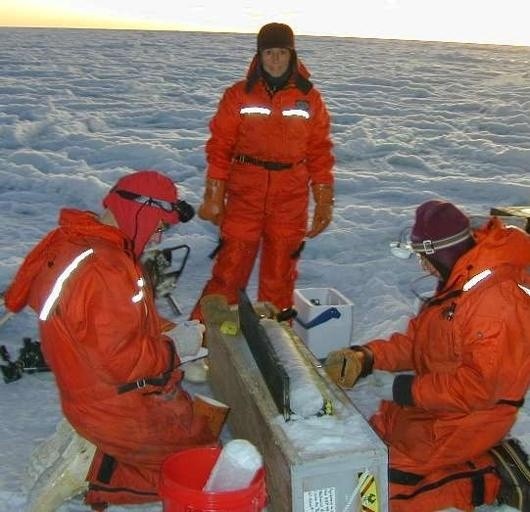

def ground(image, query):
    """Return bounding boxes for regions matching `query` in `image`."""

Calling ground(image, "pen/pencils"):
[184,322,190,328]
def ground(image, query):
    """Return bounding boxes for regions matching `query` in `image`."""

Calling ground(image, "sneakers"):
[480,438,530,512]
[18,415,102,512]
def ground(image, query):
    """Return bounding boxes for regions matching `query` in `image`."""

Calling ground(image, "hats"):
[411,197,476,280]
[248,22,311,80]
[101,165,182,261]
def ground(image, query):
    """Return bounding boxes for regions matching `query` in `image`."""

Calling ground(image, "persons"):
[329,199,529,512]
[5,173,226,511]
[179,22,334,383]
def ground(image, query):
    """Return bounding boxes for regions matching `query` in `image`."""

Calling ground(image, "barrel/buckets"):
[156,446,270,512]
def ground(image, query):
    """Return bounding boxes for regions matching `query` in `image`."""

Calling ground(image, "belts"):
[229,152,308,170]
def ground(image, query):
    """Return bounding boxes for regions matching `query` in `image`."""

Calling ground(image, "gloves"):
[161,315,206,359]
[198,174,227,225]
[306,182,335,239]
[365,369,400,404]
